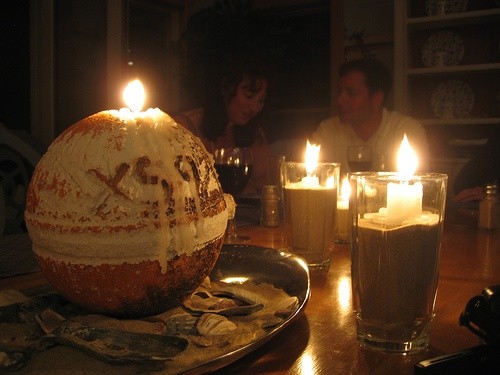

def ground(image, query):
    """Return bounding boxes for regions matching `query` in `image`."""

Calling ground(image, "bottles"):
[477,183,500,232]
[261,185,281,227]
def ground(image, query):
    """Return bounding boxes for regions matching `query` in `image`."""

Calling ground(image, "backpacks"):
[0,128,48,233]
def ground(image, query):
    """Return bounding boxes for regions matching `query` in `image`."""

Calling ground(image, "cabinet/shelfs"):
[392,0,500,126]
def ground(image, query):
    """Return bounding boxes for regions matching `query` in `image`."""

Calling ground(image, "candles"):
[283,136,338,258]
[337,177,353,241]
[355,132,444,328]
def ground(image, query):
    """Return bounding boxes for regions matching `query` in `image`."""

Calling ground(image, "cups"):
[347,145,373,171]
[278,160,341,267]
[336,200,353,243]
[347,174,448,352]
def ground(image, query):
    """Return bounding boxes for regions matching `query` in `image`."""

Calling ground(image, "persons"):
[312,59,430,200]
[177,66,307,196]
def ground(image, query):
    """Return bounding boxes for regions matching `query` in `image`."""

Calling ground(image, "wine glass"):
[215,146,252,243]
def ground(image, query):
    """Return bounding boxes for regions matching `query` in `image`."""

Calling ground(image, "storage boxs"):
[235,197,263,225]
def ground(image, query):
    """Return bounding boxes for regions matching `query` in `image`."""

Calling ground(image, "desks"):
[228,221,500,375]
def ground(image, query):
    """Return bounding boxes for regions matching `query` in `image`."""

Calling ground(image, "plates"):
[430,79,475,117]
[422,30,463,67]
